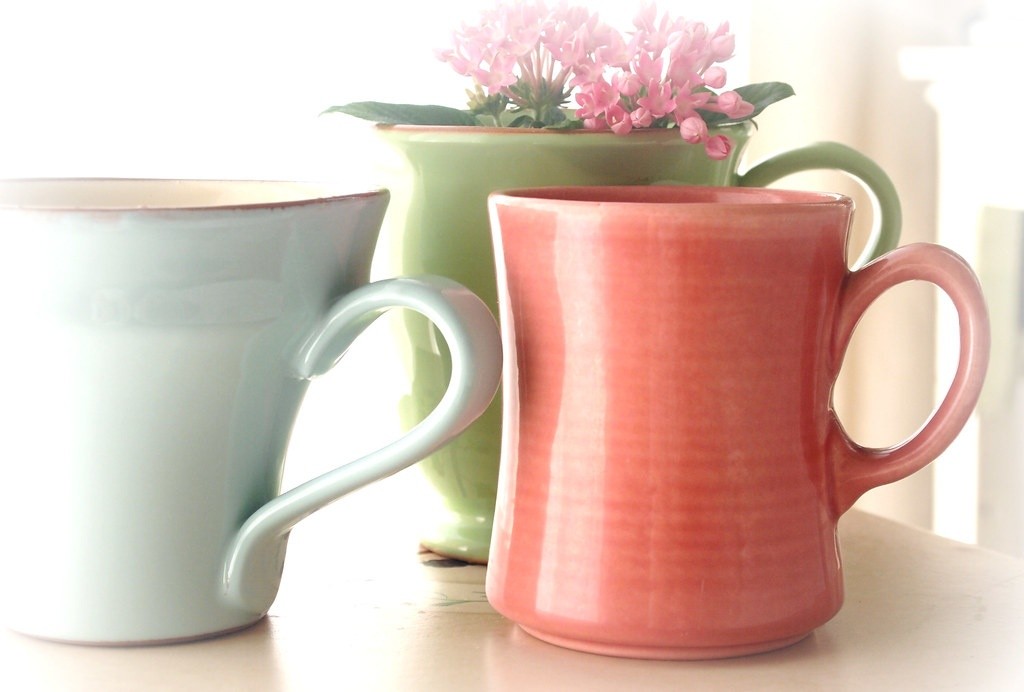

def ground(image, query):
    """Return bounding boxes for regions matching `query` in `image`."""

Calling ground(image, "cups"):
[488,188,992,661]
[0,178,501,648]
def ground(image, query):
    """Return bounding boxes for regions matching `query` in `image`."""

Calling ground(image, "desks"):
[0,509,1024,692]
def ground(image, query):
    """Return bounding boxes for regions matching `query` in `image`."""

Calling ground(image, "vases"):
[374,122,902,564]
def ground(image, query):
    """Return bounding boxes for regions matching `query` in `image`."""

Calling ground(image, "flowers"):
[319,0,796,159]
